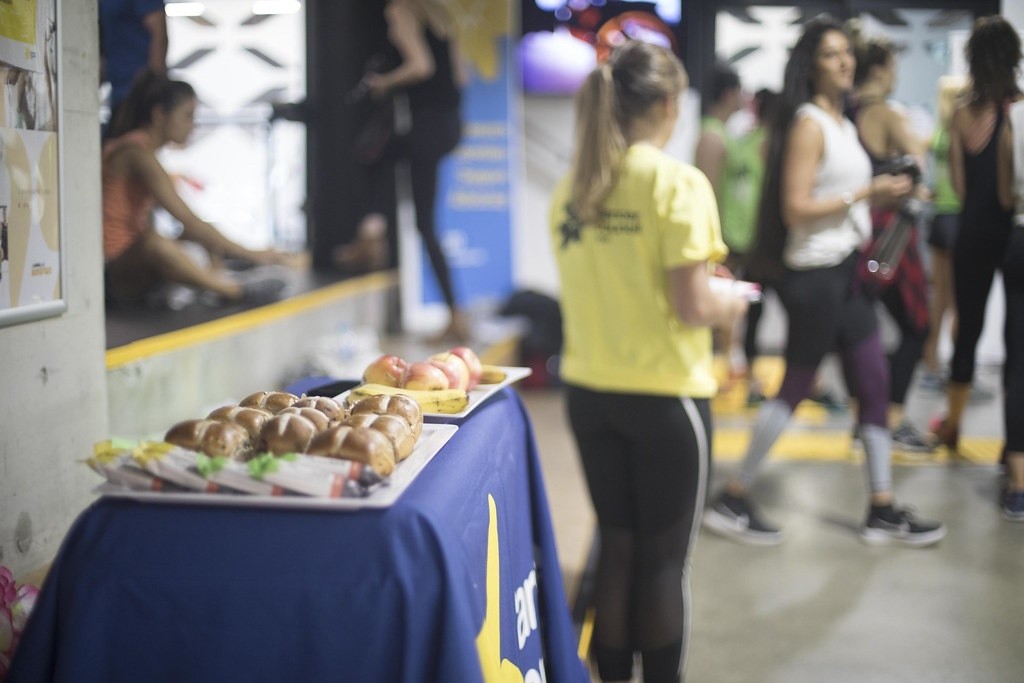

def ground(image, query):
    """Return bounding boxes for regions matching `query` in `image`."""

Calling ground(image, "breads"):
[165,393,422,475]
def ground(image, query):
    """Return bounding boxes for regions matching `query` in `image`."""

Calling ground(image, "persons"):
[102,72,309,306]
[98,0,169,137]
[548,43,749,683]
[334,0,467,343]
[692,16,1024,549]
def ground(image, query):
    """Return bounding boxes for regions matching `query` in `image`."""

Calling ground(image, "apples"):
[364,346,481,392]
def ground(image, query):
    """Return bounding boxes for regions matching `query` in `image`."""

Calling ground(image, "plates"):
[333,367,531,418]
[93,422,459,508]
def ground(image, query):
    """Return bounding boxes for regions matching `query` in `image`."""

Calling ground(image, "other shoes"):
[969,378,996,405]
[746,389,766,409]
[915,371,945,394]
[229,262,294,300]
[928,412,957,450]
[809,381,853,410]
[999,475,1024,522]
[852,417,939,452]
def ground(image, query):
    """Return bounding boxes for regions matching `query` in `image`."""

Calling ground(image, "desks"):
[4,377,593,683]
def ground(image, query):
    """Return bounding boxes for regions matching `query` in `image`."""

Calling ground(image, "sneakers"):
[701,490,784,545]
[860,505,949,547]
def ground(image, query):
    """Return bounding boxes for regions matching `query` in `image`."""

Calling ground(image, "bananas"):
[481,366,507,384]
[350,384,470,414]
